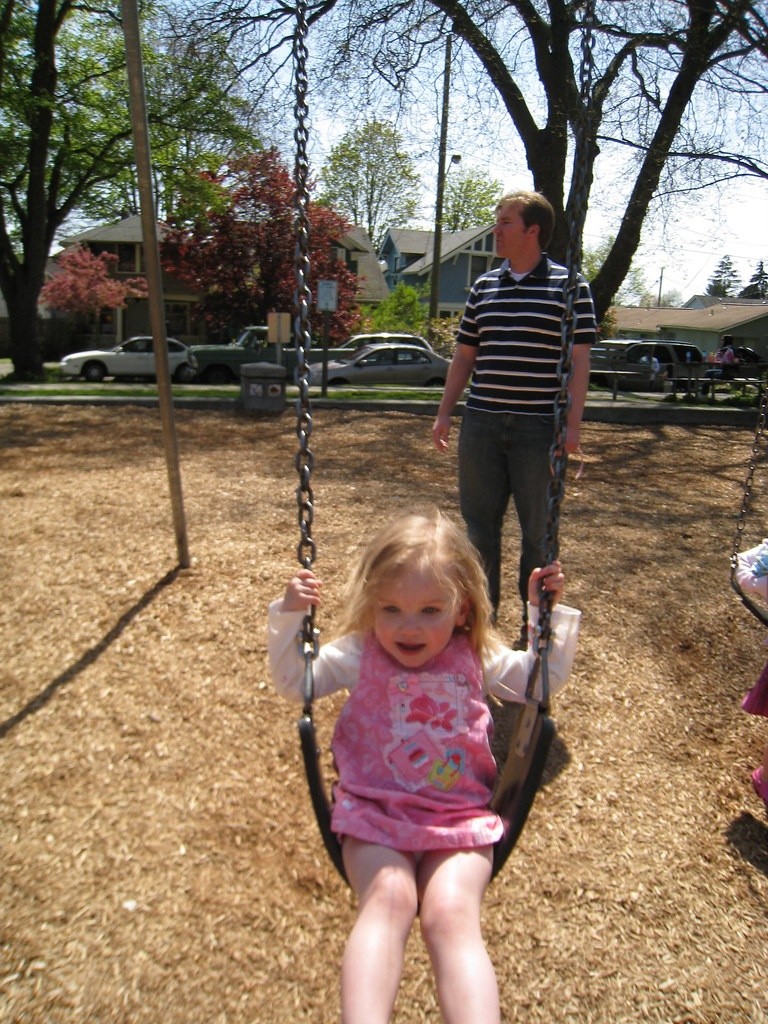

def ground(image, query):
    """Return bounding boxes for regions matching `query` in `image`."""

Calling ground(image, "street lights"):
[426,152,464,340]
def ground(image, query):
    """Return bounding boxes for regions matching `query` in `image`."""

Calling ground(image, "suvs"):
[588,341,707,391]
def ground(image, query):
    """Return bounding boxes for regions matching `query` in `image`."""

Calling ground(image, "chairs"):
[376,352,394,365]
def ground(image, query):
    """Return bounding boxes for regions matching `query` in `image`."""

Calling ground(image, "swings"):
[288,0,603,901]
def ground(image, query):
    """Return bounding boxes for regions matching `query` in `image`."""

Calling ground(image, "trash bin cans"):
[239,361,287,414]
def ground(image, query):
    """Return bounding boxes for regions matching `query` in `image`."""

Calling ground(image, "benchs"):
[588,370,643,399]
[665,378,763,401]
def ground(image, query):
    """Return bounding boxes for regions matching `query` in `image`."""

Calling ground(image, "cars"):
[338,333,436,364]
[58,333,198,384]
[302,342,453,389]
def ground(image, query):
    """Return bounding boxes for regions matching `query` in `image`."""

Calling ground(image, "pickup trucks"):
[188,324,356,385]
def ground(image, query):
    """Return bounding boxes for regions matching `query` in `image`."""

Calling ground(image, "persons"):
[640,349,659,391]
[269,517,581,1024]
[698,335,735,401]
[736,538,768,802]
[432,192,598,649]
